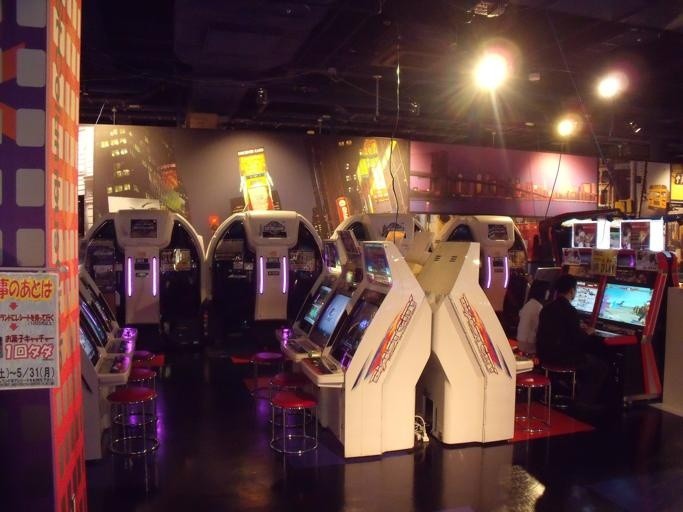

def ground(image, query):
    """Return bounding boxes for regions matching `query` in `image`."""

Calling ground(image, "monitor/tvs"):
[309,284,357,350]
[298,282,333,335]
[598,279,655,332]
[570,277,600,317]
[79,276,113,369]
[329,288,387,373]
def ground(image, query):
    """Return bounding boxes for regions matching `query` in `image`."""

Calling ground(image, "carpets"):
[507,401,595,444]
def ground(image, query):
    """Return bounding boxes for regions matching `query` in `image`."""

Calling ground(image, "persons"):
[515,280,549,353]
[536,274,609,403]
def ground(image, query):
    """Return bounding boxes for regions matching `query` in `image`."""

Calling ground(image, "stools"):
[269,390,320,453]
[248,352,285,400]
[106,387,159,457]
[519,354,542,368]
[508,339,518,350]
[515,372,553,432]
[131,350,157,403]
[537,364,576,410]
[117,369,158,440]
[269,372,311,427]
